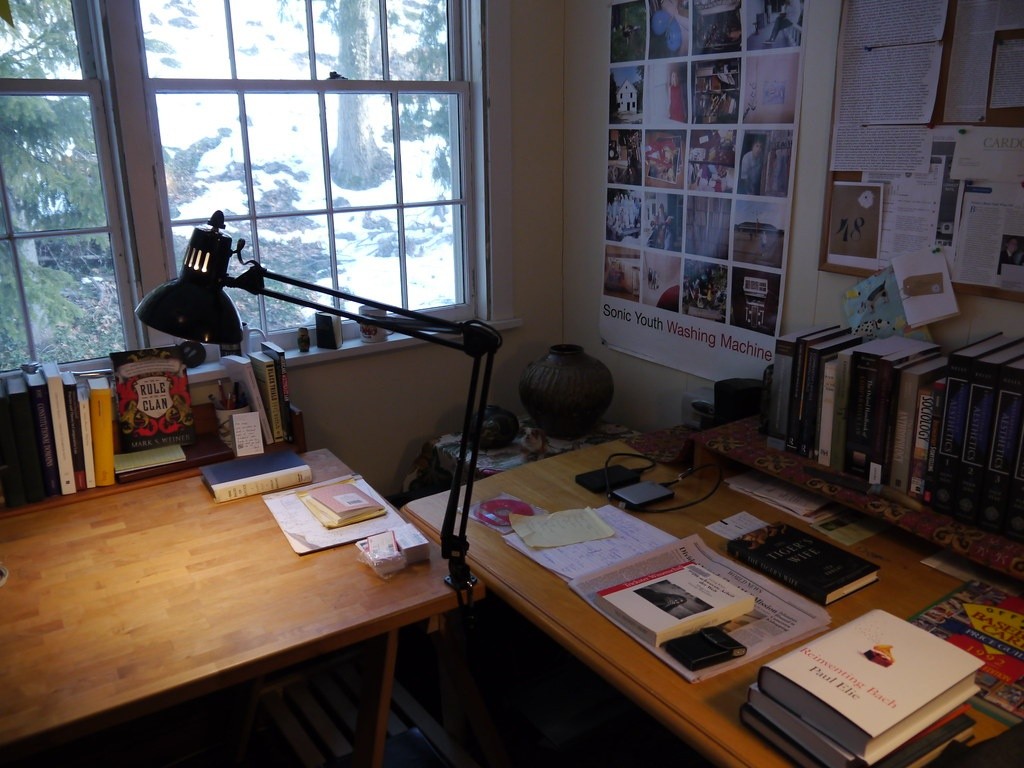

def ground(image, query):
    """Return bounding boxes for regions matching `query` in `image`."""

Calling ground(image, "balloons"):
[652,10,682,51]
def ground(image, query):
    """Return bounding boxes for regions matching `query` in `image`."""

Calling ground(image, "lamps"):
[132,211,502,627]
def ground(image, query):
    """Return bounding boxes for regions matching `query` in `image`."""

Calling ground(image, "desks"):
[402,416,644,495]
[399,425,1024,768]
[0,447,484,768]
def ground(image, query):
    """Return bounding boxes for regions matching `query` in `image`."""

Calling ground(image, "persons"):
[651,204,676,251]
[760,231,767,256]
[763,0,801,44]
[741,139,763,196]
[997,235,1020,275]
[668,70,687,122]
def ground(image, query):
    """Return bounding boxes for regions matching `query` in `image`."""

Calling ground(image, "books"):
[109,345,198,474]
[198,451,312,503]
[766,324,1024,542]
[0,360,115,510]
[739,609,985,768]
[595,560,755,648]
[262,473,407,554]
[727,520,881,606]
[222,342,295,445]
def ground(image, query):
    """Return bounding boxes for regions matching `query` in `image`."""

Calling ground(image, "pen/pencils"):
[208,378,240,409]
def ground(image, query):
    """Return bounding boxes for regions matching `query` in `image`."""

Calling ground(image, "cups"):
[220,322,266,363]
[359,306,397,342]
[216,404,250,443]
